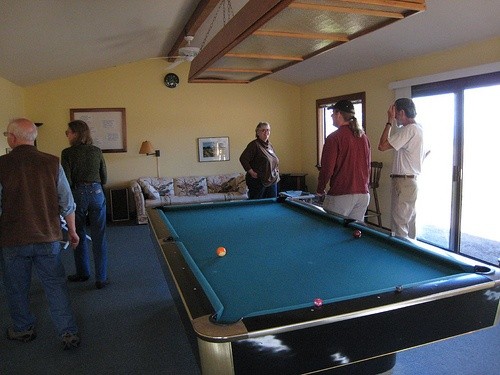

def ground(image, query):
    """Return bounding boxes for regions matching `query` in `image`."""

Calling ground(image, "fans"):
[145,36,200,71]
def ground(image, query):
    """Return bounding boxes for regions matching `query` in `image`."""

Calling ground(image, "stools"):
[364,161,383,227]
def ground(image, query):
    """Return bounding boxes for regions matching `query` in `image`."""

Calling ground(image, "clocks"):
[164,73,179,88]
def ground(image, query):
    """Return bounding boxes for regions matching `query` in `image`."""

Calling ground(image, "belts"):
[392,175,414,178]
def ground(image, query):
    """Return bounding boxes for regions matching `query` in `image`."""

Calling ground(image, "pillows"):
[138,179,159,200]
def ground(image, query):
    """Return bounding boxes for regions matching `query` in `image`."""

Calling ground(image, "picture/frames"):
[70,108,127,153]
[197,137,230,162]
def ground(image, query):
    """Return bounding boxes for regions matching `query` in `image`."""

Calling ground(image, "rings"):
[70,241,71,243]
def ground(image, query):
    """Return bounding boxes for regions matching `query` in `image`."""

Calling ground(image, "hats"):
[327,100,354,114]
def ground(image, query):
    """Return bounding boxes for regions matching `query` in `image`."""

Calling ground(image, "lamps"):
[138,140,160,158]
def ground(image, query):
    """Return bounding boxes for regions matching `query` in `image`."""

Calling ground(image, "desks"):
[278,173,308,192]
[145,196,500,375]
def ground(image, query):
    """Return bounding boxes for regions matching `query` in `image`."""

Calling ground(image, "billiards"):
[313,298,323,307]
[352,230,362,238]
[216,246,226,256]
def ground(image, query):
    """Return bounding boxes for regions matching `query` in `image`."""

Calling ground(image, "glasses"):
[66,131,75,137]
[258,129,271,133]
[4,132,13,137]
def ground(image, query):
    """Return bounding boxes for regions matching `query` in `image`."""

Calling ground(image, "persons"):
[61,120,110,288]
[317,99,371,222]
[0,118,81,349]
[239,122,281,199]
[378,98,424,240]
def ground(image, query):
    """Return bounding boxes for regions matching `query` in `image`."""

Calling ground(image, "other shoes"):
[96,278,111,290]
[67,273,89,281]
[61,330,80,349]
[6,325,36,342]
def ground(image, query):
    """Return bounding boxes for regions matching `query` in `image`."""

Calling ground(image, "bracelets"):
[387,123,391,125]
[316,193,323,196]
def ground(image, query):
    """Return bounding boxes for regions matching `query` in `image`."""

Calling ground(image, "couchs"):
[129,174,249,224]
